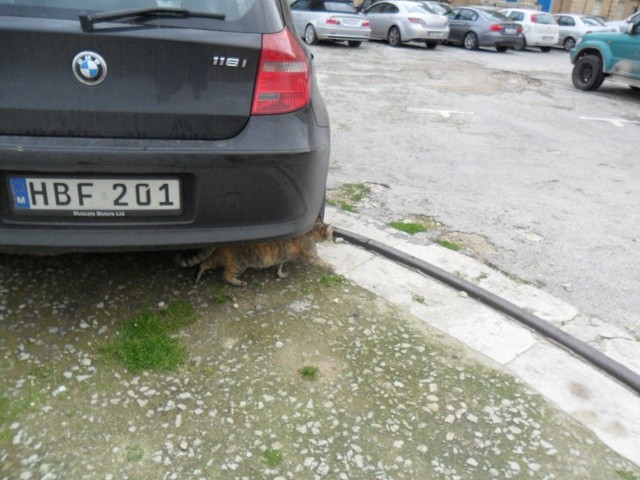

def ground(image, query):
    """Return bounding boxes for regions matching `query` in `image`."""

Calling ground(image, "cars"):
[498,8,561,52]
[605,10,640,32]
[0,0,334,260]
[553,13,621,53]
[444,6,525,53]
[570,20,640,91]
[288,0,373,48]
[360,1,452,50]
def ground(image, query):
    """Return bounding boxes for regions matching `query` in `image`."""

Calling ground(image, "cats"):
[175,216,332,288]
